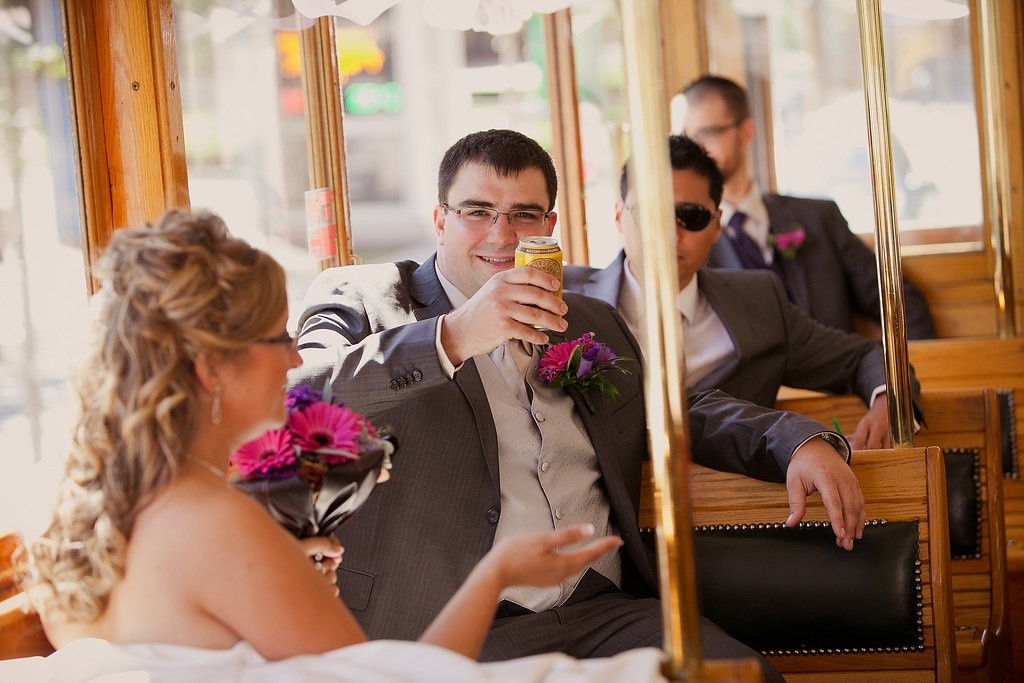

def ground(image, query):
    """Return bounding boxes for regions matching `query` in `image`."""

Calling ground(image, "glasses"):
[622,203,717,233]
[227,331,300,359]
[686,120,745,145]
[442,203,550,231]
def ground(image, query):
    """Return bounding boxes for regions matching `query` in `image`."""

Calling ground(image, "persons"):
[11,208,625,664]
[287,127,869,683]
[562,132,930,451]
[667,73,938,340]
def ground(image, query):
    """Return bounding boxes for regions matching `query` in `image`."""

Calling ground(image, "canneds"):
[514,236,563,331]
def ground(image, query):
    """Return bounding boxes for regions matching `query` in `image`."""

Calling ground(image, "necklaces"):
[187,454,231,489]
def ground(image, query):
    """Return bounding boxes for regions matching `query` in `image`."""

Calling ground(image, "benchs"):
[0,223,1024,683]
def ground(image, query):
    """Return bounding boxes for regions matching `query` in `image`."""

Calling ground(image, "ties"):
[728,211,767,269]
[489,339,531,407]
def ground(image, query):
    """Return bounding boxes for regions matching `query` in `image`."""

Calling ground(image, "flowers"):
[765,226,808,262]
[527,330,636,415]
[227,386,398,569]
